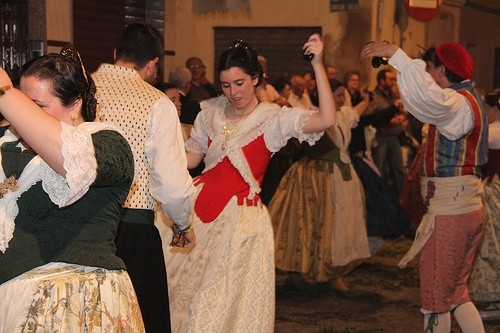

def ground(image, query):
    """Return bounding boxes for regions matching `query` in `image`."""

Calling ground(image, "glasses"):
[188,64,202,69]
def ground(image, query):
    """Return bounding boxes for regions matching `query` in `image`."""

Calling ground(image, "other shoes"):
[286,278,314,295]
[330,287,355,299]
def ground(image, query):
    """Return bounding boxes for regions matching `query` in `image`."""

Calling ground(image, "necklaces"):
[221,100,259,150]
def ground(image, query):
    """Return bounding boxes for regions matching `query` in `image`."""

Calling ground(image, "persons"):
[90,22,196,333]
[468,87,500,323]
[0,57,422,240]
[160,33,337,333]
[0,44,145,333]
[359,41,489,333]
[266,78,373,298]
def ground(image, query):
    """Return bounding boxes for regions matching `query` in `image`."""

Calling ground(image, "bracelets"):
[0,85,12,95]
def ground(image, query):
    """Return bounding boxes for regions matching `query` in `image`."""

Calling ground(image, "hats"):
[436,42,474,80]
